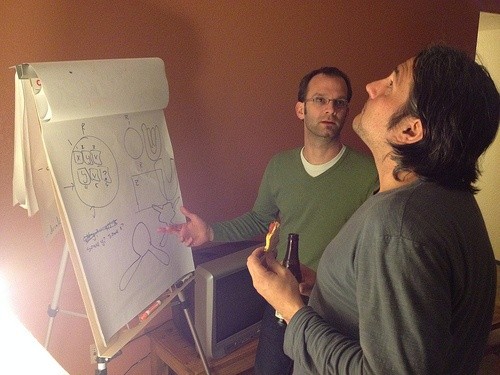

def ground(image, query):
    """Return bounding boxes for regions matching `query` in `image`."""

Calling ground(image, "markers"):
[176,272,193,288]
[140,300,162,320]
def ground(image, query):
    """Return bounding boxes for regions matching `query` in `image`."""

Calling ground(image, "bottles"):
[274,233,302,326]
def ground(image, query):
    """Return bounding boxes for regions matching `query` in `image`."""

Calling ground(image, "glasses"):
[306,96,347,108]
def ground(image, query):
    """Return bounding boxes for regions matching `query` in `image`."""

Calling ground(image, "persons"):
[155,67,380,375]
[246,41,500,375]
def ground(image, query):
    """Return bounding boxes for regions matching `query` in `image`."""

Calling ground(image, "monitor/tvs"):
[172,239,268,360]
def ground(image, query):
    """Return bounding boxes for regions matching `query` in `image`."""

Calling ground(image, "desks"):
[144,320,260,375]
